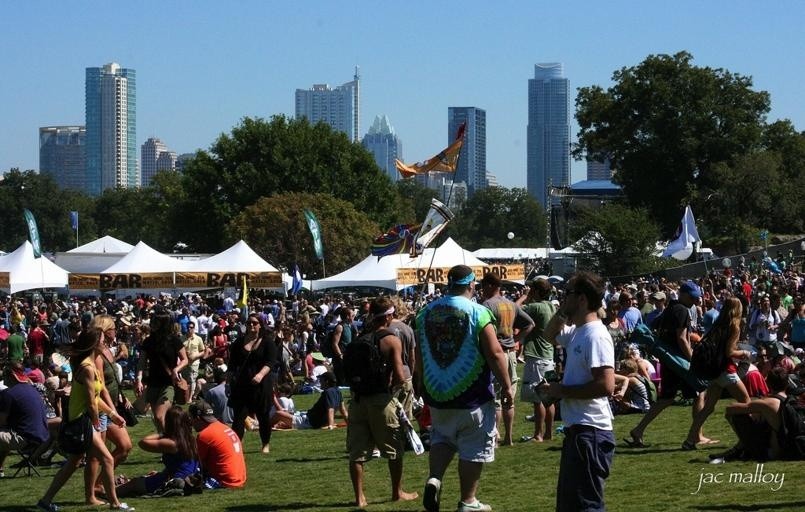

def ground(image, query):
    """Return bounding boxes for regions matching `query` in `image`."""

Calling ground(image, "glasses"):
[563,290,574,297]
[247,321,259,326]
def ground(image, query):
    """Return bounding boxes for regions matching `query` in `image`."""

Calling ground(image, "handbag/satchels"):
[115,400,138,426]
[175,373,189,405]
[56,412,94,454]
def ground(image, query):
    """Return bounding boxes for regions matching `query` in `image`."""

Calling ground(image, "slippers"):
[623,437,650,448]
[699,438,720,445]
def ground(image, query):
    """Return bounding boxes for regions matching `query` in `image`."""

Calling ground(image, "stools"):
[14,438,53,478]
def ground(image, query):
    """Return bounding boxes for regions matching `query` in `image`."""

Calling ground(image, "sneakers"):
[458,500,492,512]
[37,500,61,512]
[708,446,745,462]
[423,477,442,511]
[110,502,136,512]
[682,440,698,450]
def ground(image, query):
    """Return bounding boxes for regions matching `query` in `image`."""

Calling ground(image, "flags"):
[291,261,304,296]
[409,194,456,259]
[664,205,699,258]
[373,222,421,256]
[70,211,78,232]
[395,123,464,178]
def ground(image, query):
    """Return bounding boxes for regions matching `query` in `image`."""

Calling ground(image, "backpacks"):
[343,329,396,404]
[766,394,805,461]
[685,322,729,381]
[628,376,658,406]
[646,299,691,358]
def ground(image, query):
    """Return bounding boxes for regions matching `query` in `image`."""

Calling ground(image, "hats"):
[653,291,666,301]
[189,401,218,423]
[5,360,28,383]
[316,372,336,383]
[311,352,326,361]
[680,282,701,299]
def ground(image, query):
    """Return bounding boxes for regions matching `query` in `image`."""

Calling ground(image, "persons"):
[533,274,618,511]
[618,248,805,290]
[342,295,421,507]
[36,328,138,511]
[95,406,199,497]
[0,289,804,472]
[187,398,248,490]
[83,313,132,505]
[413,264,515,510]
